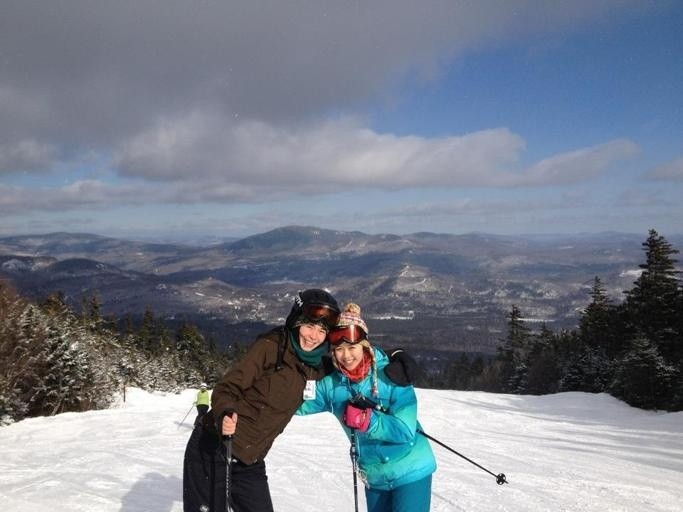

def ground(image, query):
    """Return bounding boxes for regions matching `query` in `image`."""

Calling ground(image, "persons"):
[180,288,420,512]
[192,382,210,430]
[291,302,438,512]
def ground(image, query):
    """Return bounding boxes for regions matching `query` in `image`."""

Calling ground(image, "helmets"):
[199,383,207,389]
[284,288,339,335]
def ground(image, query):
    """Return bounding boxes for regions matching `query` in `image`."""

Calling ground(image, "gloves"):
[342,400,373,433]
[384,349,418,383]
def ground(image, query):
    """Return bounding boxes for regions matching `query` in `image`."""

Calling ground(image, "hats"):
[330,303,369,352]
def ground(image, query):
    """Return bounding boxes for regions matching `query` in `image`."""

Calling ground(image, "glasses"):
[326,324,367,345]
[303,303,342,327]
